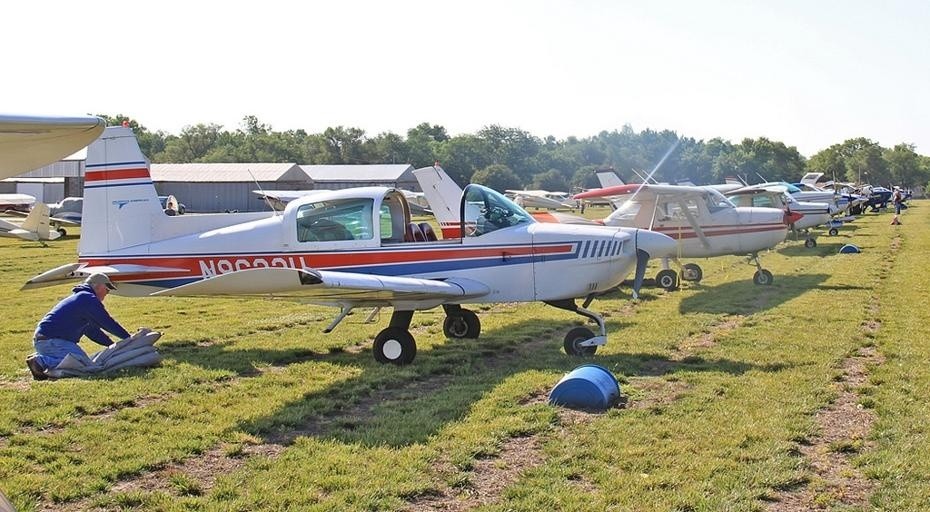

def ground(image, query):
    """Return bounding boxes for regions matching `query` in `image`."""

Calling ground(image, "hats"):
[85,273,118,290]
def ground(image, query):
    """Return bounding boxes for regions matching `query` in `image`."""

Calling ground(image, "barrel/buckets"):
[547,364,621,411]
[839,243,860,253]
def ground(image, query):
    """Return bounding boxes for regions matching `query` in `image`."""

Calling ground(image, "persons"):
[26,272,128,380]
[165,203,176,216]
[892,186,902,214]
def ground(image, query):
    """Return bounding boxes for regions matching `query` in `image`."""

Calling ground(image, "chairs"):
[405,221,438,243]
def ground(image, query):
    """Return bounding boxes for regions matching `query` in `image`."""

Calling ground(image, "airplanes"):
[20,125,673,363]
[48,195,186,237]
[0,193,60,240]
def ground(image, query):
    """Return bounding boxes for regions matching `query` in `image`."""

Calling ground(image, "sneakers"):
[26,355,49,380]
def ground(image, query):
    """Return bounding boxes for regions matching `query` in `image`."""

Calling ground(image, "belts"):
[34,336,56,340]
